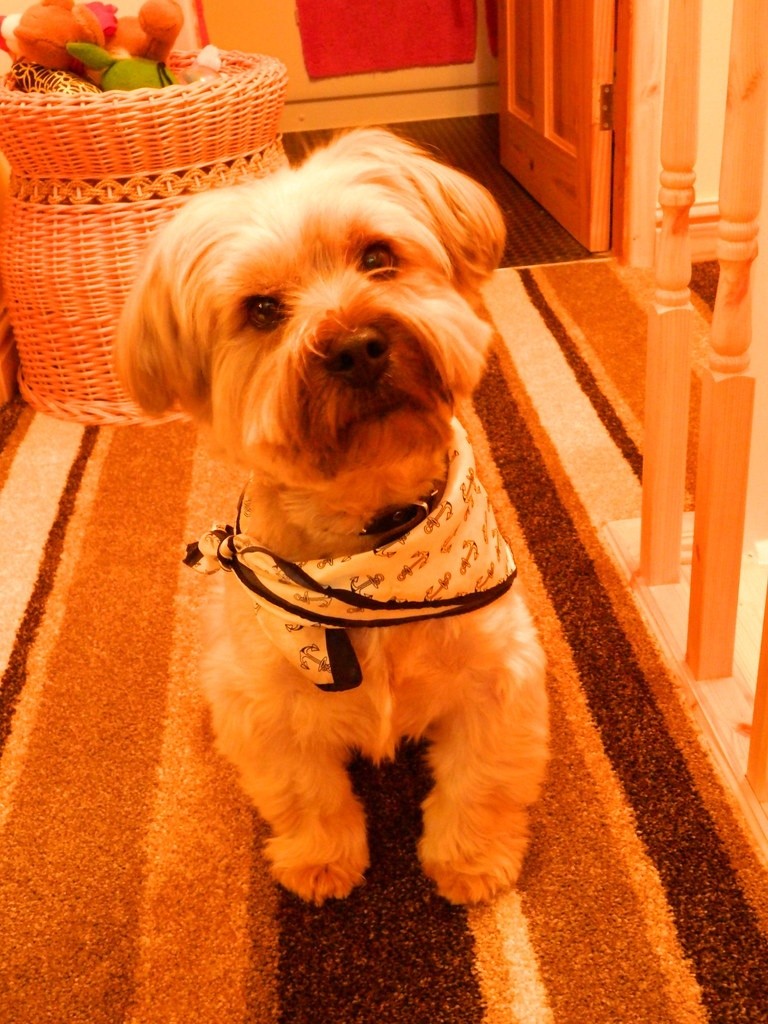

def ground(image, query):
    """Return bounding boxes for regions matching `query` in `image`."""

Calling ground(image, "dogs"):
[112,128,547,909]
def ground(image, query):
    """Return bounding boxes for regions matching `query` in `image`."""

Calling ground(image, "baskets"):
[0,30,292,427]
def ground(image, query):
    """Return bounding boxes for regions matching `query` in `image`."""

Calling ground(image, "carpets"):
[0,251,768,1024]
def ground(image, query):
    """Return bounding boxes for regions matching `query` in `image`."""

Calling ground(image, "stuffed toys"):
[0,2,222,90]
